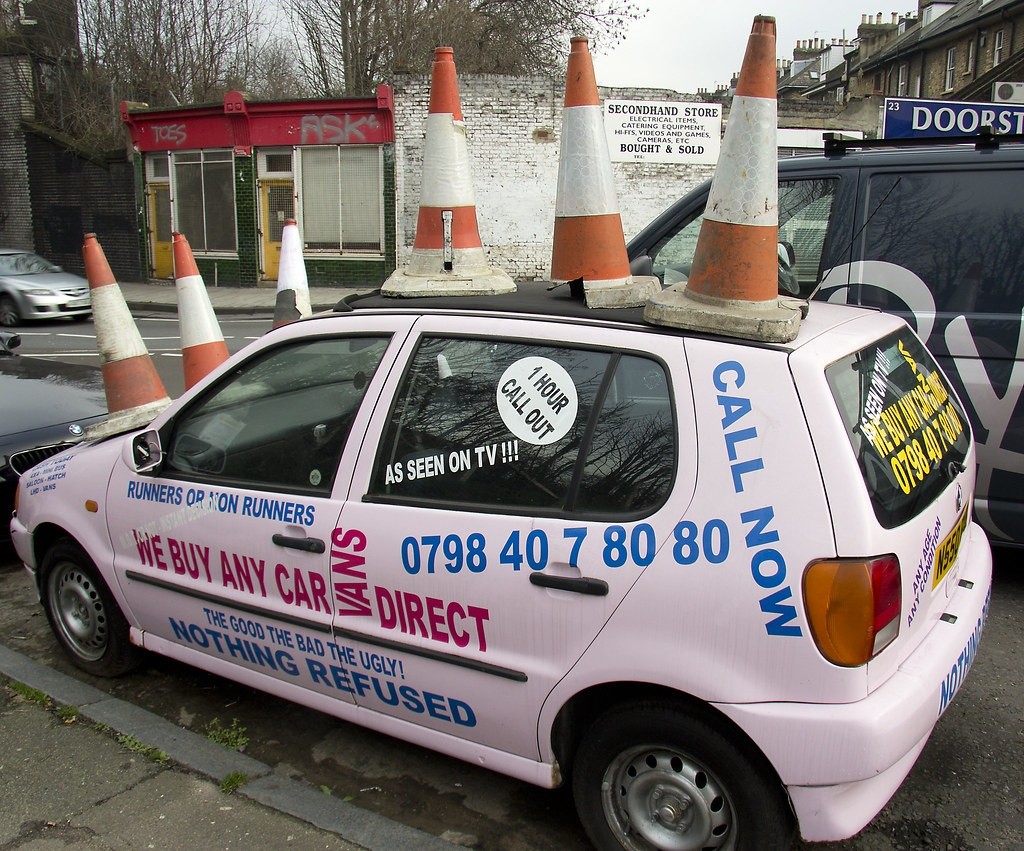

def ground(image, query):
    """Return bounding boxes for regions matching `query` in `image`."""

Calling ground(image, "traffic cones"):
[264,218,321,331]
[170,229,274,412]
[381,46,517,299]
[78,232,178,441]
[642,15,812,344]
[544,34,663,310]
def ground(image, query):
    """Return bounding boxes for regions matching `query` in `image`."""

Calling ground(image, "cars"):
[1,330,109,572]
[8,277,997,851]
[0,248,93,326]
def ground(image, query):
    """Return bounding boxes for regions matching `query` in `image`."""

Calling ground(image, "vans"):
[628,98,1024,553]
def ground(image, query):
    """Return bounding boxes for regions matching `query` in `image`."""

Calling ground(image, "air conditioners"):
[991,81,1024,103]
